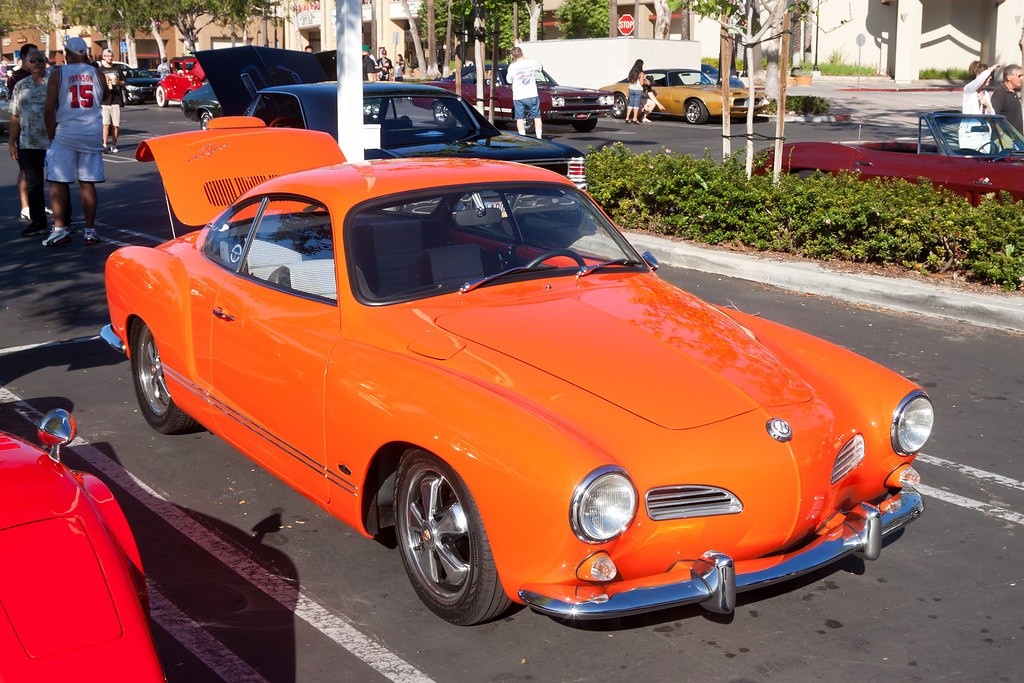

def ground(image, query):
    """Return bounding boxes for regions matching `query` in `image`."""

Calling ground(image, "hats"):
[647,76,656,86]
[62,37,87,56]
[362,45,370,51]
[382,50,386,55]
[102,49,113,57]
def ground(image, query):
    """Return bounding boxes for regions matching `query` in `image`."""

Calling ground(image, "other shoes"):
[44,207,53,215]
[102,144,107,153]
[21,207,32,220]
[642,118,652,123]
[110,146,119,154]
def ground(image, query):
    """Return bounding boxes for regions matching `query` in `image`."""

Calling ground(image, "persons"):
[0,37,125,247]
[363,45,405,102]
[625,59,665,125]
[505,47,543,139]
[157,57,170,80]
[990,64,1024,152]
[958,61,1001,154]
[305,45,314,52]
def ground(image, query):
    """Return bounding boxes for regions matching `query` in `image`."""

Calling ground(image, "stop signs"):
[618,14,635,35]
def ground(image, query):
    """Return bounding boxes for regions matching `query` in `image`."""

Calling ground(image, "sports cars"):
[0,406,168,683]
[98,113,938,625]
[750,107,1024,209]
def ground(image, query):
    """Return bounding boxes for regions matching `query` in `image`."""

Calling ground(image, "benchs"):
[381,127,473,146]
[364,114,415,131]
[211,218,455,296]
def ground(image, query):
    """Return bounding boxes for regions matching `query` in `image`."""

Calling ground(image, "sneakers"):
[22,223,49,235]
[42,230,72,247]
[84,228,98,245]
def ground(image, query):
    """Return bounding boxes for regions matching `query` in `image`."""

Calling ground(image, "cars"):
[0,60,15,135]
[596,68,771,126]
[410,64,616,135]
[700,65,745,90]
[111,55,223,130]
[189,45,588,224]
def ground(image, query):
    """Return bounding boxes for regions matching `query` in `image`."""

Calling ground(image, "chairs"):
[410,244,498,288]
[266,259,376,302]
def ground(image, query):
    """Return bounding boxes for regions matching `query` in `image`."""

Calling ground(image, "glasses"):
[29,58,44,63]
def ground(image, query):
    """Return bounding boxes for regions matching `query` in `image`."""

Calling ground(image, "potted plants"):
[793,57,814,87]
[766,94,837,123]
[776,55,796,87]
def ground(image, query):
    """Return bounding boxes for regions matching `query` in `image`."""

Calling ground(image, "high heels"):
[625,120,630,123]
[632,120,640,123]
